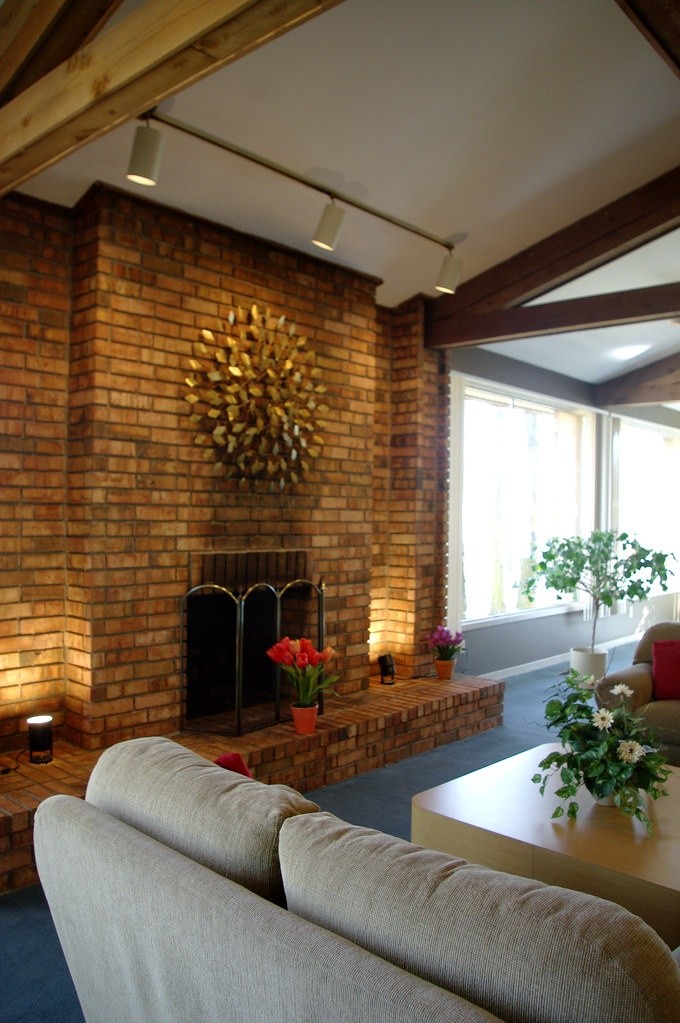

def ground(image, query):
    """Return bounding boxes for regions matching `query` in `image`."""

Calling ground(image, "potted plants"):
[521,528,677,691]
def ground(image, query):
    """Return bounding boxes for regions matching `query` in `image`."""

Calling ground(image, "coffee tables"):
[411,742,680,952]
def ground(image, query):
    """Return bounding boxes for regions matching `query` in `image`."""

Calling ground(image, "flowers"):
[424,626,465,659]
[531,667,674,836]
[266,636,343,706]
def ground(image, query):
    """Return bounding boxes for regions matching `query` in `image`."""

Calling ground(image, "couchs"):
[34,736,680,1022]
[595,620,680,771]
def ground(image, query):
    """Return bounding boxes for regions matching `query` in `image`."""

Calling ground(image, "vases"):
[593,788,617,807]
[288,701,319,735]
[432,658,457,680]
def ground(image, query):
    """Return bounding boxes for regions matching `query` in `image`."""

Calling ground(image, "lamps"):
[377,654,397,684]
[435,245,461,294]
[310,193,347,250]
[26,715,55,763]
[127,105,165,186]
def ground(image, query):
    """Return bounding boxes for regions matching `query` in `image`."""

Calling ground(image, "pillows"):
[651,639,680,701]
[213,752,253,779]
